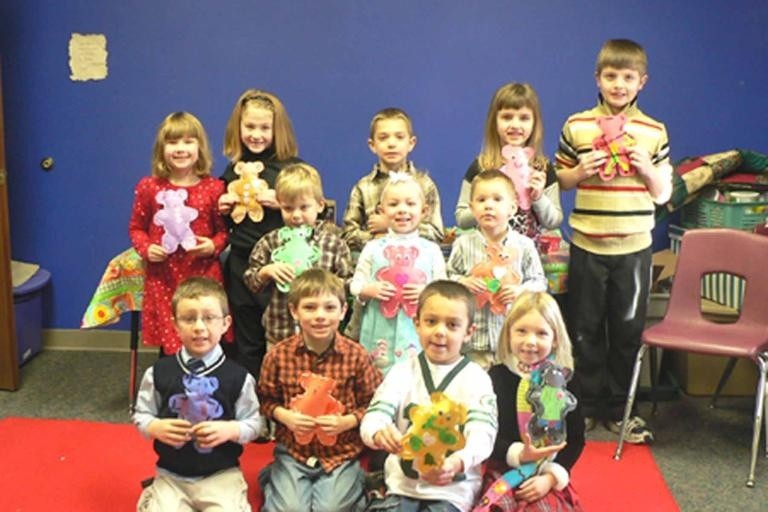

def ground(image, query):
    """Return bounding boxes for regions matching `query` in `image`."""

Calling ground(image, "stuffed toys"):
[592,112,637,181]
[471,242,520,315]
[152,189,199,254]
[525,361,578,445]
[499,144,536,213]
[374,245,427,318]
[272,225,321,292]
[226,160,268,225]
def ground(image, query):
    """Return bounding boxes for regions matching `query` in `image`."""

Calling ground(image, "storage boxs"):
[11,259,51,367]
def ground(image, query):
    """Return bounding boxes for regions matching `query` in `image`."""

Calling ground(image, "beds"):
[79,225,745,417]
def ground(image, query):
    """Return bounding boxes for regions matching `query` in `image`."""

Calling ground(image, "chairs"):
[613,228,768,490]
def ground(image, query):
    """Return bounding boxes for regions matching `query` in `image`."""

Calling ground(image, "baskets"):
[680,195,768,232]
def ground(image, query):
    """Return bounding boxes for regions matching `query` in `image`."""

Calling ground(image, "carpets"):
[0,416,683,512]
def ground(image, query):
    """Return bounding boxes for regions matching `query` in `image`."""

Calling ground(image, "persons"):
[349,169,446,472]
[255,268,384,510]
[358,281,500,511]
[131,279,269,511]
[217,88,300,378]
[479,289,586,512]
[243,161,353,444]
[343,107,446,342]
[128,111,235,358]
[454,82,564,248]
[553,40,674,445]
[446,169,548,372]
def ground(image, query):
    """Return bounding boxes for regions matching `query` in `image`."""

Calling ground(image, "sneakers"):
[364,469,385,490]
[604,415,654,445]
